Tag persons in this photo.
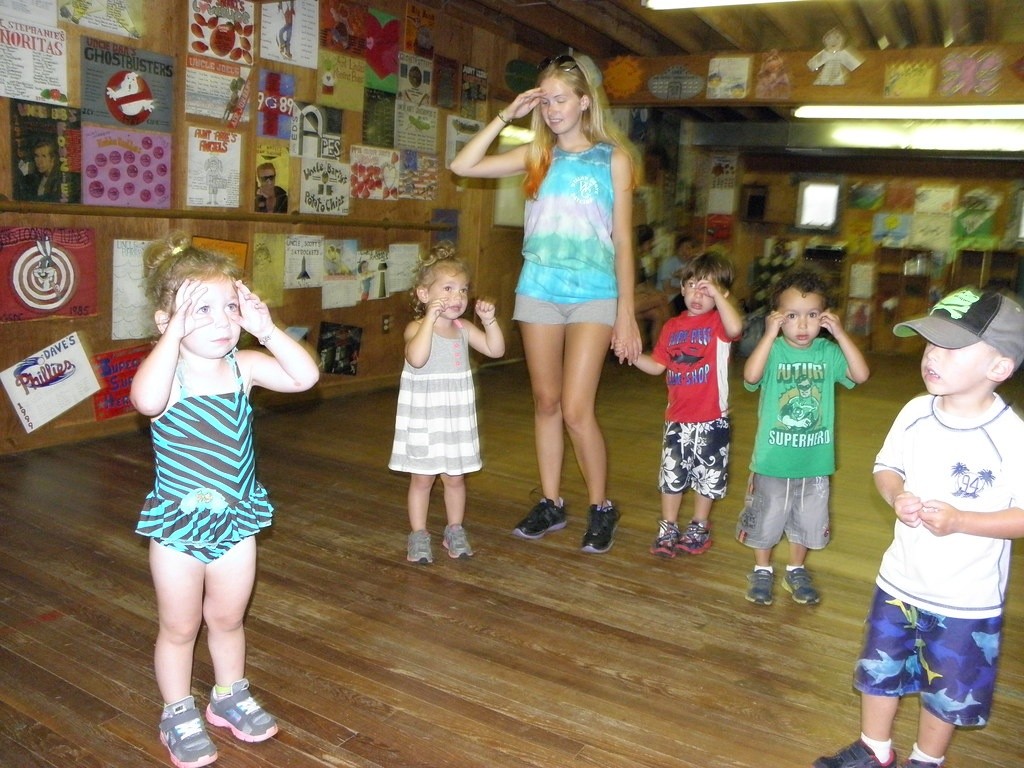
[18,139,81,203]
[734,271,869,608]
[633,224,671,349]
[449,47,642,553]
[813,286,1024,768]
[387,239,505,563]
[614,250,742,557]
[131,234,319,768]
[657,235,699,317]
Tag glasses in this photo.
[538,55,585,80]
[262,175,274,180]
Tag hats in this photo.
[892,289,1024,366]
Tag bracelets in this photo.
[256,324,275,345]
[497,110,510,124]
[481,317,496,326]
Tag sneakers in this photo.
[811,738,897,768]
[676,519,712,554]
[205,678,278,742]
[650,519,679,557]
[745,570,773,606]
[582,500,620,553]
[782,568,821,605]
[159,696,218,768]
[407,529,433,564]
[513,489,567,539]
[443,524,474,559]
